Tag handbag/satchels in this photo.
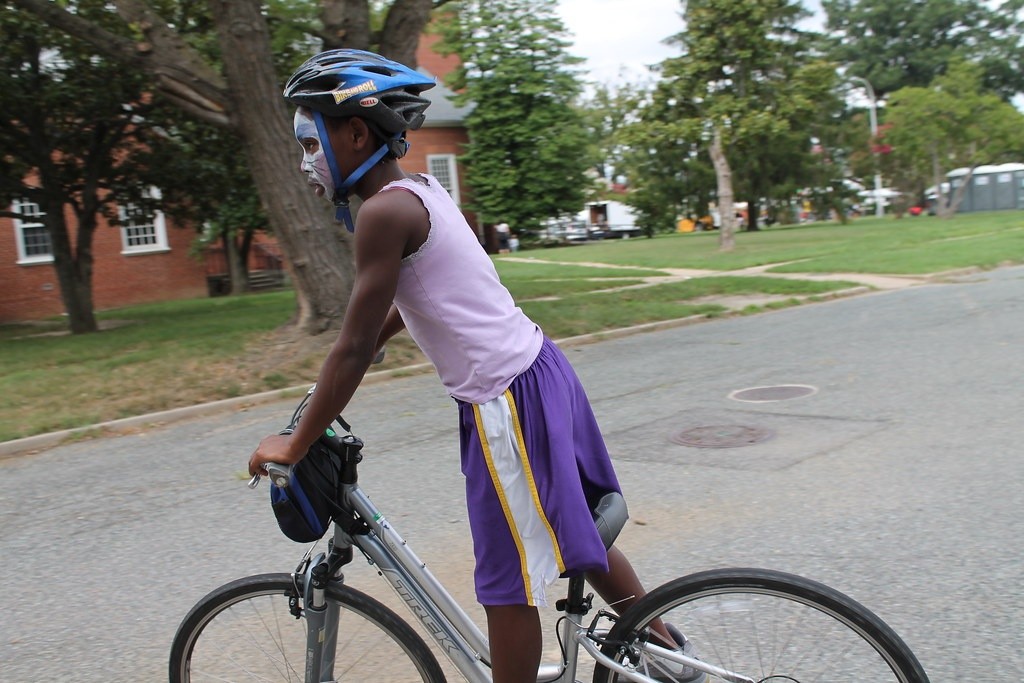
[270,417,372,544]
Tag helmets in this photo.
[281,48,436,139]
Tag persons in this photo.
[246,45,711,683]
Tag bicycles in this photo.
[167,384,929,683]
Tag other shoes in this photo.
[618,623,709,683]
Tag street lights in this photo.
[841,77,886,218]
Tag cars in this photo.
[565,223,588,240]
[854,187,898,216]
[832,179,865,198]
[588,224,644,241]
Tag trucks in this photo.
[584,201,643,230]
